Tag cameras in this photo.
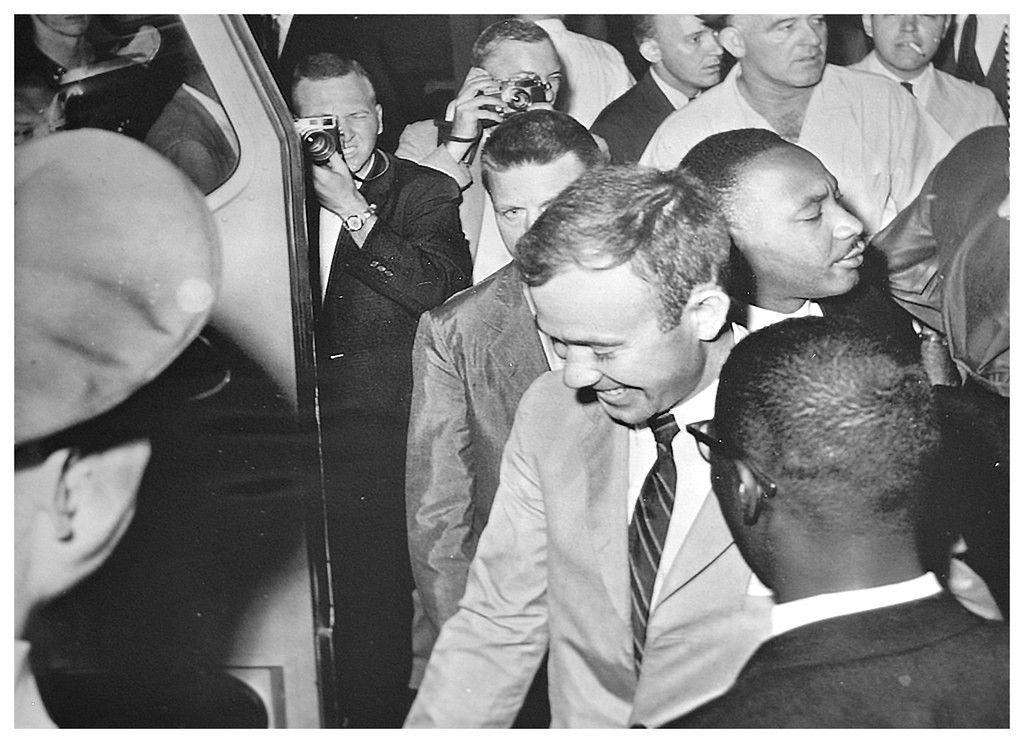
[478,77,540,125]
[293,115,341,163]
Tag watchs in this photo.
[342,204,376,231]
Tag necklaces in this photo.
[405,110,604,695]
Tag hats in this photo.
[15,127,231,444]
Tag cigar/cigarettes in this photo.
[909,42,925,57]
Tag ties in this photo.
[627,410,682,681]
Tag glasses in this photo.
[685,419,777,497]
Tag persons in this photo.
[636,15,957,245]
[285,51,472,729]
[141,40,239,195]
[14,129,234,729]
[674,128,921,366]
[868,124,1009,397]
[514,14,636,132]
[656,316,1010,729]
[840,13,1008,143]
[588,16,723,163]
[397,164,1004,729]
[395,19,613,285]
